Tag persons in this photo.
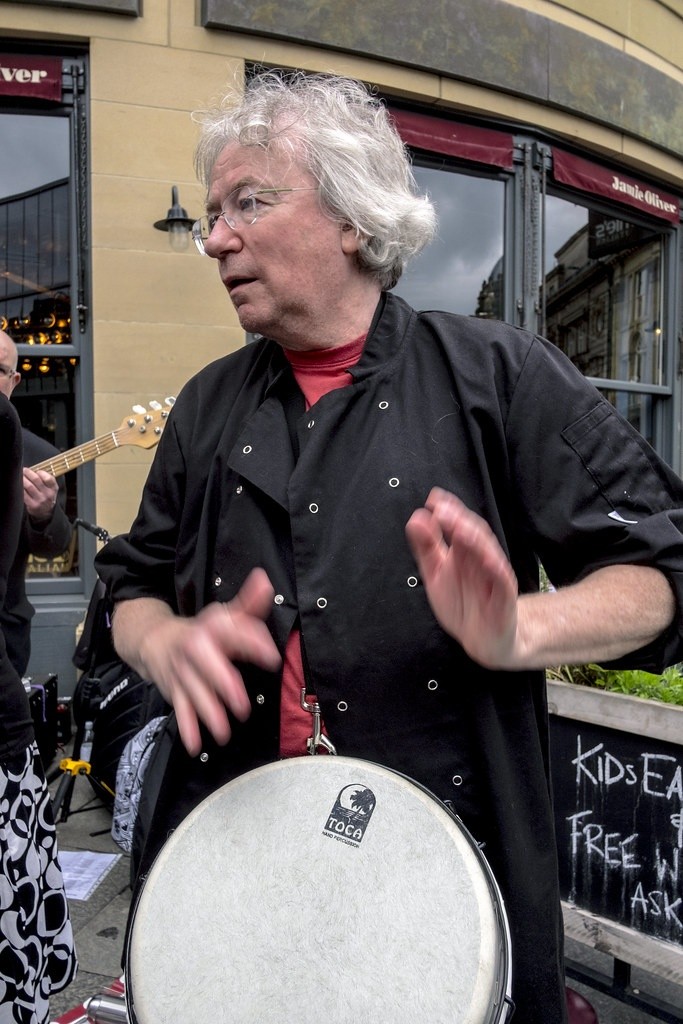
[69,64,683,1024]
[0,320,96,1014]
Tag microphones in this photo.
[76,518,108,541]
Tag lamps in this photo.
[154,186,197,253]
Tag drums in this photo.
[123,755,518,1024]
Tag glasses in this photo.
[190,186,319,256]
[0,364,15,377]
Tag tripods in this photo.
[46,579,118,825]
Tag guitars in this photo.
[23,394,180,479]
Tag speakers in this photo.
[19,671,57,772]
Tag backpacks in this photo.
[111,716,169,854]
